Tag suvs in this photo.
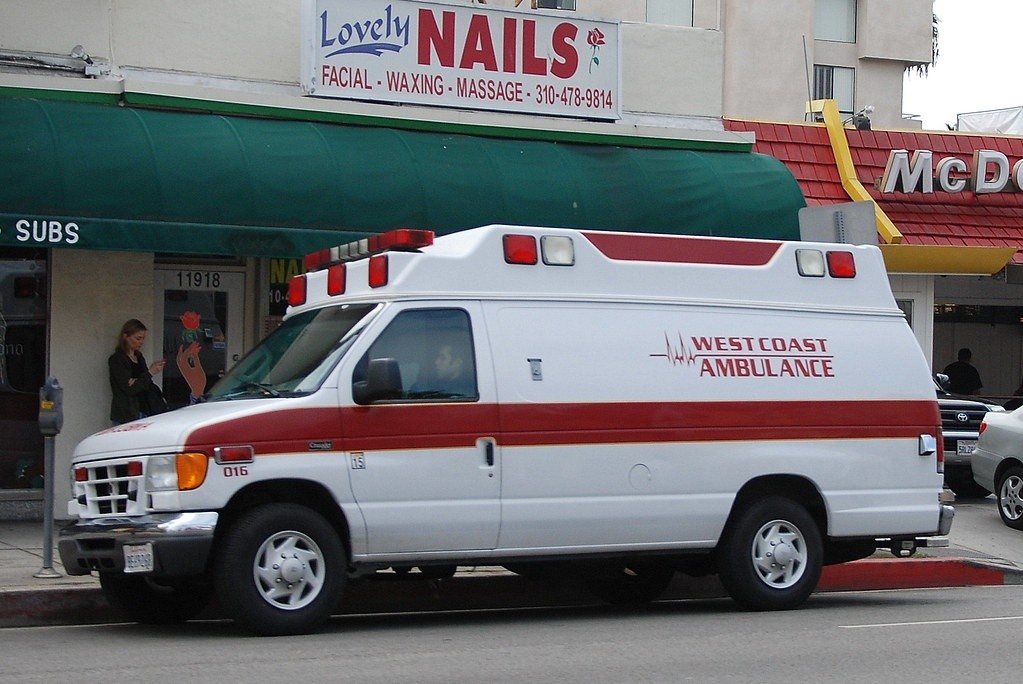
[931,372,1009,497]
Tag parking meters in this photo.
[34,375,66,579]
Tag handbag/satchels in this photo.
[139,384,167,417]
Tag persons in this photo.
[108,319,169,425]
[409,343,476,399]
[942,348,983,397]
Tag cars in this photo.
[969,403,1023,530]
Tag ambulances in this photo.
[60,221,956,638]
